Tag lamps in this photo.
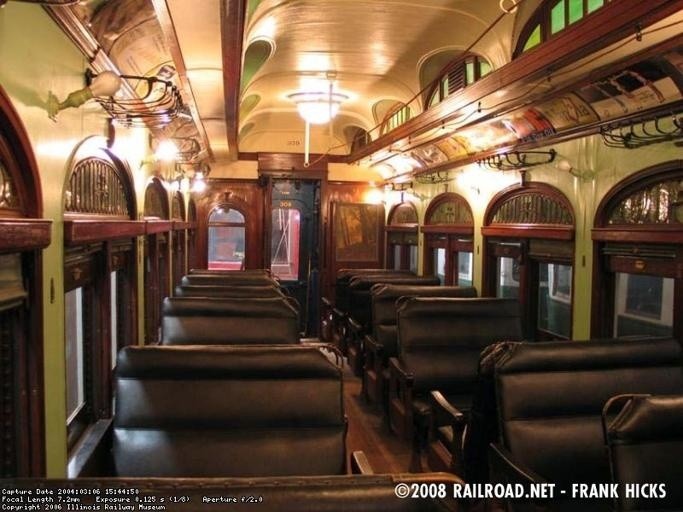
[47,71,120,122]
[406,188,425,200]
[287,91,347,125]
[141,142,178,167]
[557,161,591,182]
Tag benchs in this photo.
[0,270,683,512]
